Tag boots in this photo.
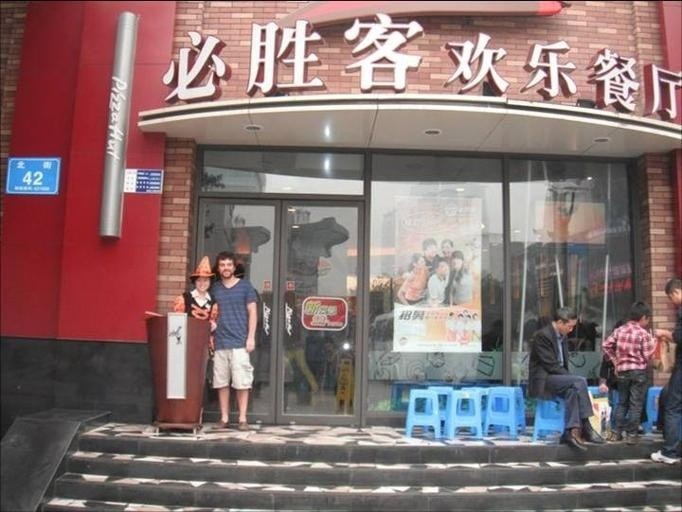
[610,429,641,444]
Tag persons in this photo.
[650,277,681,464]
[529,306,606,453]
[211,251,260,430]
[599,320,645,435]
[600,302,659,446]
[396,237,477,310]
[173,256,219,430]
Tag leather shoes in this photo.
[582,428,605,443]
[213,419,228,430]
[560,436,589,454]
[238,421,247,432]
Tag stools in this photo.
[642,388,663,434]
[406,386,619,441]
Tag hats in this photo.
[189,255,215,279]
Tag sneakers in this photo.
[651,447,679,464]
